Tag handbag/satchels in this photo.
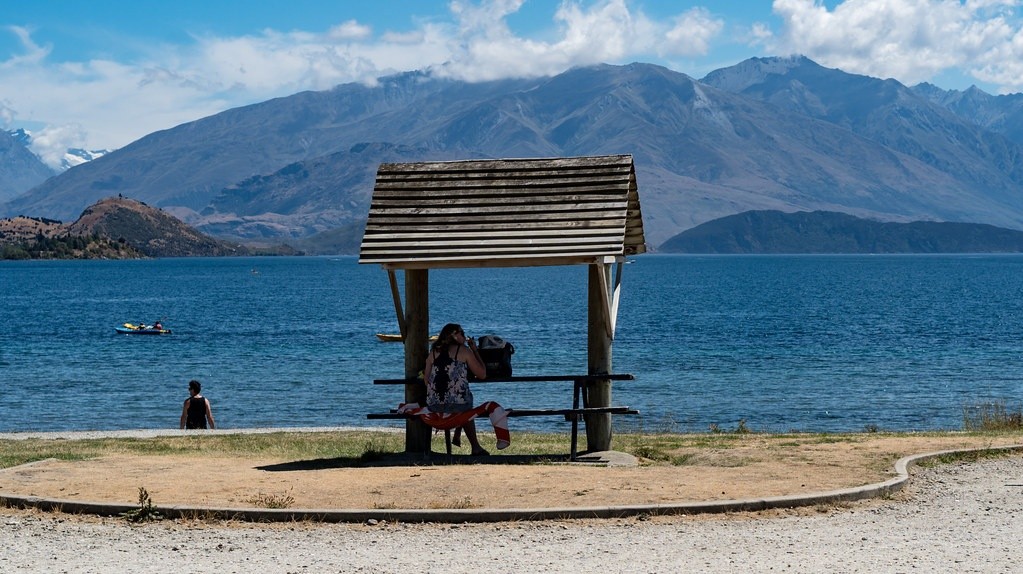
[467,335,515,379]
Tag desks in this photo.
[373,374,636,463]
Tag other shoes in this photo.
[451,439,461,447]
[470,450,489,456]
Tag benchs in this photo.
[366,406,629,421]
[388,408,640,415]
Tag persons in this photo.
[181,381,214,429]
[136,323,145,330]
[153,322,162,330]
[424,323,490,455]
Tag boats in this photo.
[114,324,171,335]
[377,333,439,341]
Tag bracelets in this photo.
[474,350,478,352]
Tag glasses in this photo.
[458,330,464,336]
